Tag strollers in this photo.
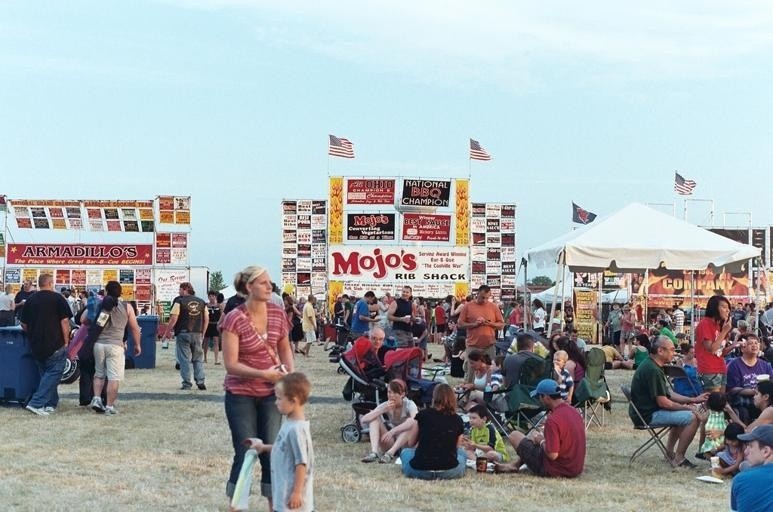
[335,332,444,444]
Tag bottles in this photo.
[484,383,492,402]
[162,336,168,352]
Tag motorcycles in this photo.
[56,319,81,385]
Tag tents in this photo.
[524,202,761,339]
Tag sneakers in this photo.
[25,405,55,417]
[198,383,206,390]
[181,386,191,390]
[91,396,105,412]
[360,451,378,462]
[104,407,119,415]
[380,451,394,463]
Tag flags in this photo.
[571,201,596,226]
[673,170,695,195]
[327,134,354,158]
[469,139,493,161]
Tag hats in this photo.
[621,304,630,311]
[737,425,773,446]
[181,282,193,290]
[529,379,561,399]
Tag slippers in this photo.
[661,452,700,469]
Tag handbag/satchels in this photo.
[67,324,88,359]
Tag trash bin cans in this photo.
[0,327,41,407]
[126,315,158,369]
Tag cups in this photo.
[476,456,487,472]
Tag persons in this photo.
[0,279,247,415]
[730,423,773,512]
[604,294,771,480]
[269,282,588,479]
[239,372,314,512]
[214,265,293,512]
